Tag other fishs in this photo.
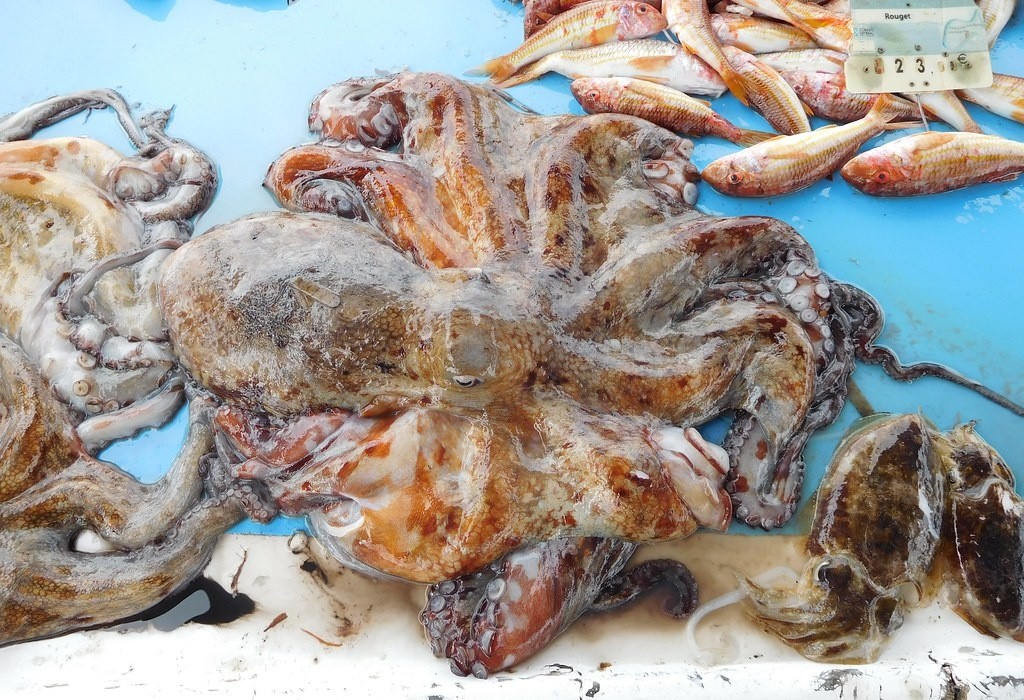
[473,1,1024,198]
